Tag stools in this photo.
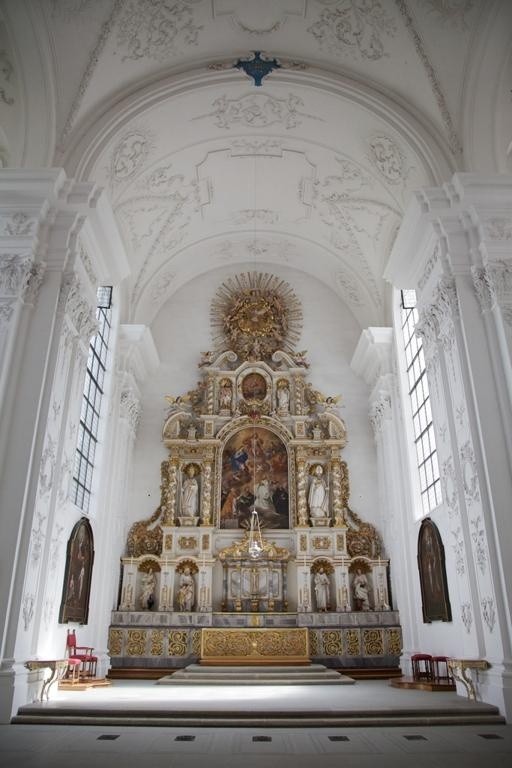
[431,656,454,684]
[411,654,433,683]
[67,658,81,679]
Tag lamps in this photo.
[247,340,264,558]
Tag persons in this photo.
[314,566,331,614]
[137,566,157,611]
[351,567,373,612]
[220,432,281,518]
[177,469,199,515]
[217,383,233,409]
[420,528,441,600]
[275,383,288,412]
[177,568,196,613]
[63,524,91,613]
[306,465,331,517]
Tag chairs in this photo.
[68,629,98,678]
